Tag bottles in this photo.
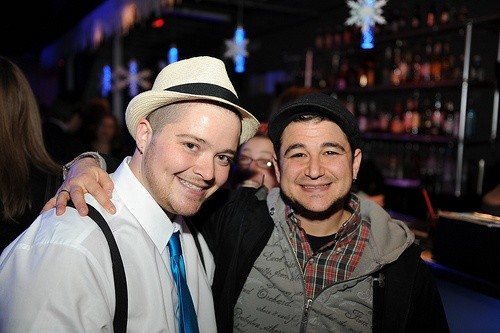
[310,7,483,191]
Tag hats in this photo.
[267,92,362,141]
[124,56,261,146]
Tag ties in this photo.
[167,230,200,333]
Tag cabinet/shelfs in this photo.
[304,18,500,223]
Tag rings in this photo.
[59,188,71,197]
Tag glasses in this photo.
[237,154,274,169]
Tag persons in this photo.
[229,130,279,195]
[0,55,263,333]
[38,84,124,173]
[38,83,452,333]
[0,57,65,253]
